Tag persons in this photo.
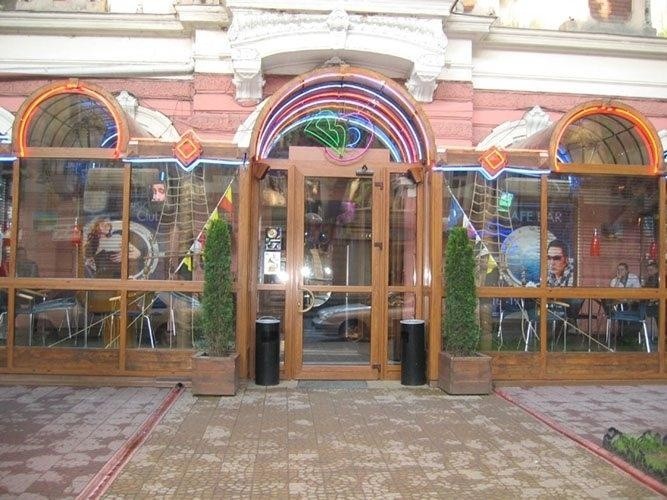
[546,238,574,287]
[149,183,166,202]
[609,262,641,288]
[84,213,141,280]
[642,262,659,288]
[14,247,39,278]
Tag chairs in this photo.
[491,285,659,352]
[0,288,155,347]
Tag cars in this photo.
[313,291,404,344]
[15,291,200,350]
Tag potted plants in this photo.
[190,217,240,398]
[438,228,496,397]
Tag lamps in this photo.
[589,206,601,255]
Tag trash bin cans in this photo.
[400,319,426,385]
[255,315,280,386]
